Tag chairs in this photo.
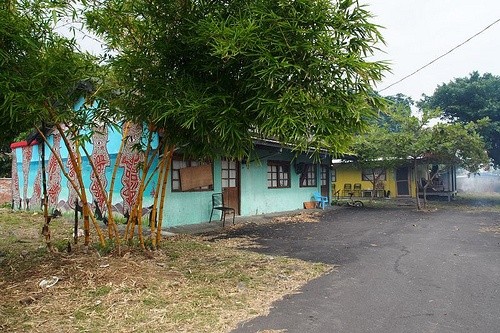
[314,191,329,210]
[208,192,235,226]
[341,183,352,197]
[353,183,362,196]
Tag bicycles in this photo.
[333,190,364,207]
